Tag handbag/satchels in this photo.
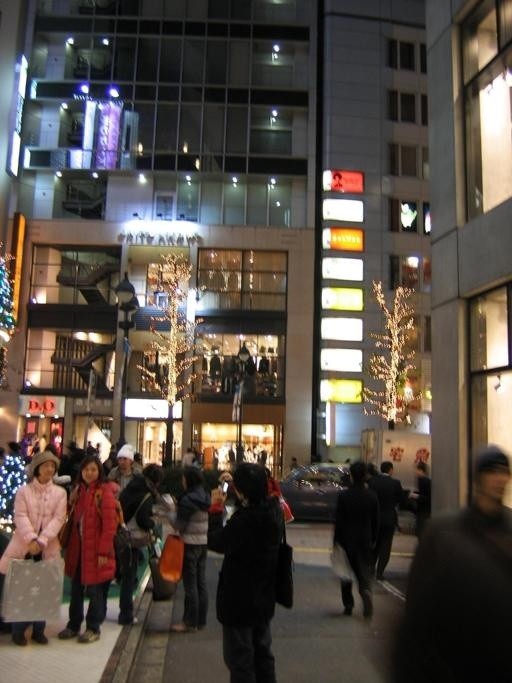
[113,523,137,586]
[277,541,293,608]
[1,557,65,622]
[159,533,184,583]
[126,517,155,547]
[58,510,72,546]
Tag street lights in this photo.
[115,272,144,446]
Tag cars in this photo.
[274,461,352,520]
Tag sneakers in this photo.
[58,627,79,638]
[78,628,100,642]
[12,634,26,644]
[170,623,197,632]
[30,631,47,643]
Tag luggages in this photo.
[147,544,177,597]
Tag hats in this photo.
[116,443,134,460]
[31,450,59,475]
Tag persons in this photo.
[333,460,407,619]
[414,460,433,537]
[0,439,209,647]
[207,461,285,682]
[289,457,299,471]
[394,446,511,681]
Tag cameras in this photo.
[221,480,229,496]
